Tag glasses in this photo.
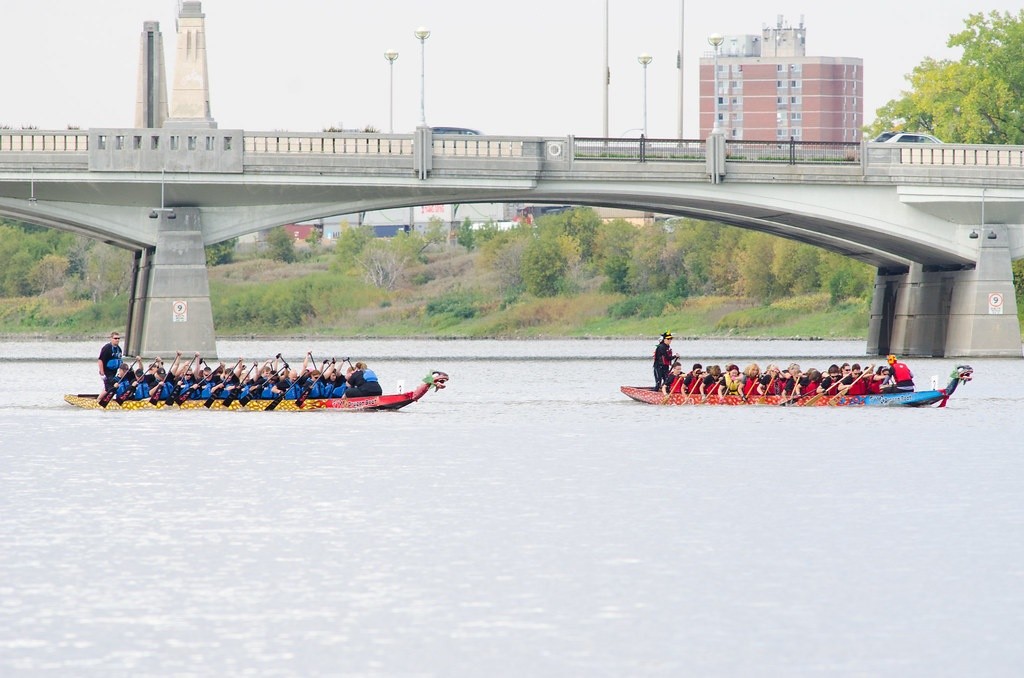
[854,372,861,376]
[883,373,891,377]
[844,368,851,372]
[794,375,798,380]
[112,337,121,341]
[185,373,194,378]
[830,374,839,379]
[314,376,318,379]
[203,372,209,378]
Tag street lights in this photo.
[708,33,725,134]
[638,53,653,147]
[385,48,399,135]
[415,27,431,125]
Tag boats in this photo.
[620,365,974,408]
[64,370,449,412]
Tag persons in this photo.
[112,351,382,400]
[883,355,915,393]
[97,332,122,400]
[653,332,680,392]
[662,362,889,403]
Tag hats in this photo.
[660,330,677,339]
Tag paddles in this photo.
[98,348,356,412]
[659,353,878,408]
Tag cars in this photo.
[432,127,480,135]
[873,131,943,143]
